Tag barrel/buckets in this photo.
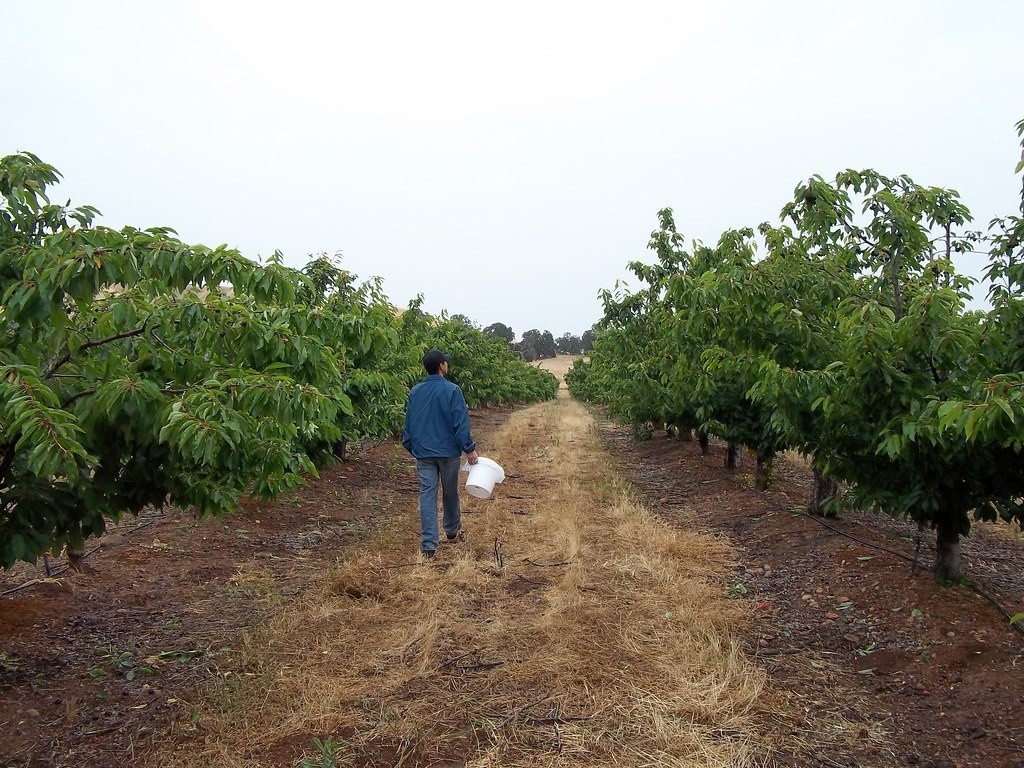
[465,458,504,499]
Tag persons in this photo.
[400,350,478,557]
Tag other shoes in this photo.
[447,521,461,541]
[422,550,435,558]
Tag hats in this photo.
[422,350,450,372]
